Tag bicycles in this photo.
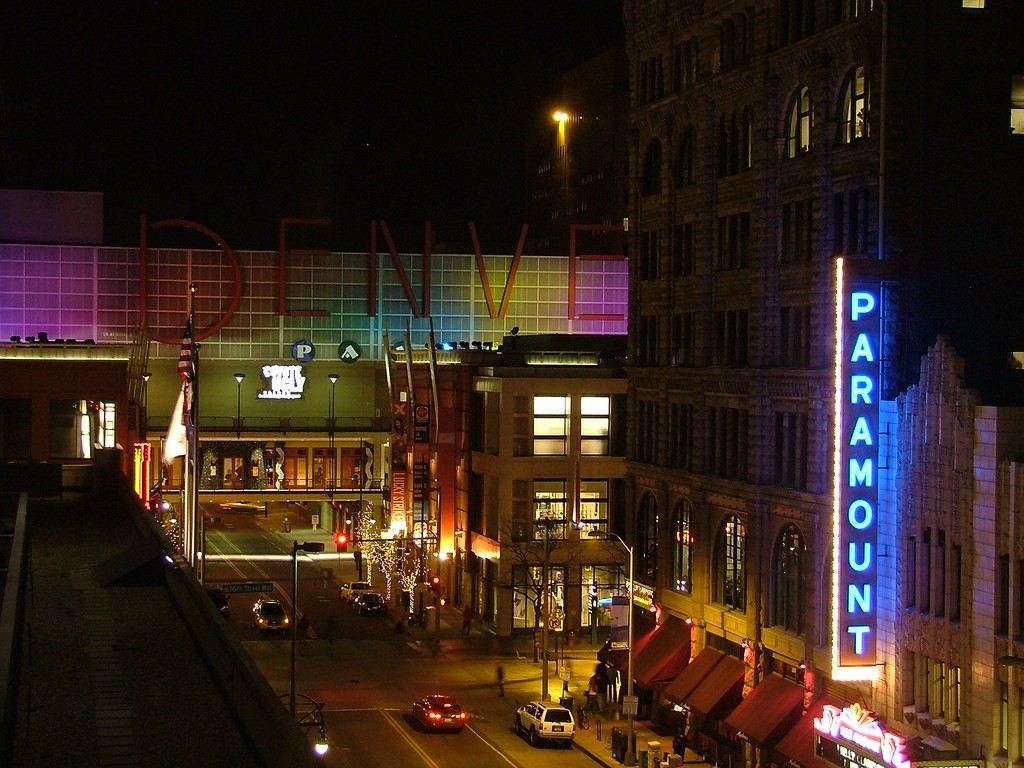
[574,704,590,731]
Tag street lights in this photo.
[327,373,341,436]
[233,372,246,439]
[288,539,326,721]
[140,370,153,437]
[587,528,637,766]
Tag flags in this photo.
[164,309,198,464]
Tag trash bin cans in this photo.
[638,749,648,768]
[648,740,662,768]
[612,726,636,763]
[558,697,574,713]
[564,629,578,647]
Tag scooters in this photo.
[407,609,430,628]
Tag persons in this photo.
[536,570,540,579]
[314,466,323,484]
[297,612,309,644]
[354,550,362,570]
[672,728,688,763]
[621,586,629,599]
[394,418,406,436]
[595,663,619,712]
[495,666,505,697]
[515,598,525,617]
[460,606,473,635]
[393,617,408,641]
[540,511,598,532]
[556,570,561,580]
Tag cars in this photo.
[220,501,266,514]
[413,694,466,734]
[340,581,375,605]
[252,597,291,635]
[353,593,388,616]
[205,588,232,621]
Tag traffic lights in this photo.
[433,576,441,588]
[335,532,349,553]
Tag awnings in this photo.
[597,612,849,768]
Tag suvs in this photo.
[515,701,576,748]
